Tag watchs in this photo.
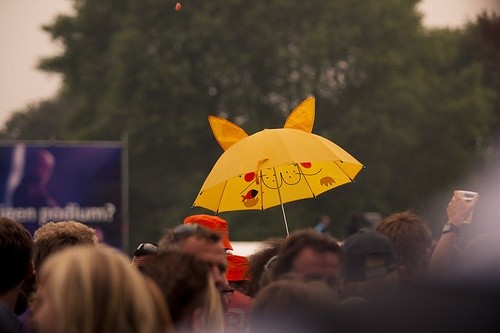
[440,223,460,235]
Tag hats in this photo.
[342,229,396,279]
[225,254,252,281]
[184,215,233,251]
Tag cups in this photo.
[454,190,476,225]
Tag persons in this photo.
[10,146,61,207]
[0,189,500,333]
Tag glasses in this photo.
[137,243,157,253]
[220,288,234,296]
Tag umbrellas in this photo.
[192,97,363,237]
